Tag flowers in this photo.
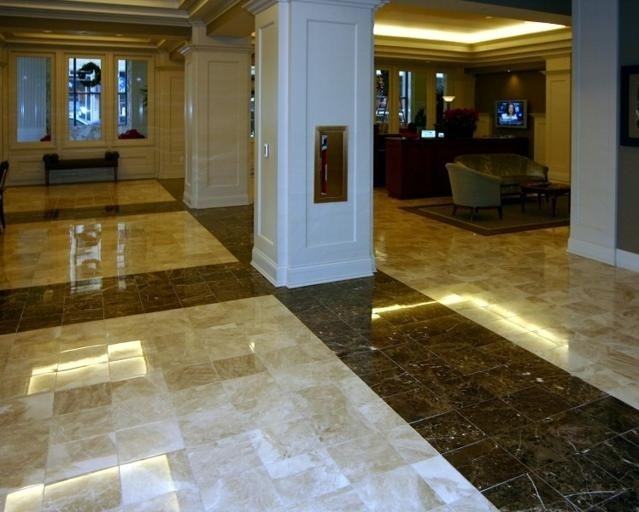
[442,108,480,122]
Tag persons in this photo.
[501,103,518,121]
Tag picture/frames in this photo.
[620,65,639,146]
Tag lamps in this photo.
[442,95,456,110]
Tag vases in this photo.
[447,119,474,139]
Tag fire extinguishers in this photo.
[321,133,329,196]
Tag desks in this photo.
[45,159,118,186]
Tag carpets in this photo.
[397,193,570,236]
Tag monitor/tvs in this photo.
[493,99,527,128]
[420,129,437,138]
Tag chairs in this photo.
[444,153,548,220]
[0,160,10,229]
